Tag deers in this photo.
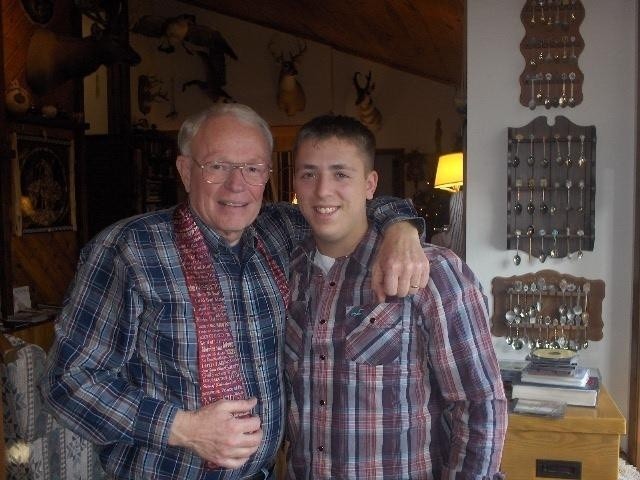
[352,70,384,132]
[273,35,308,118]
[24,0,142,96]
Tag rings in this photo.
[410,285,419,288]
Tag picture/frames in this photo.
[9,132,80,238]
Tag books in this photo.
[17,309,49,323]
[520,361,591,387]
[511,372,600,407]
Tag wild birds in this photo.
[157,11,197,53]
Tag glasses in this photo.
[189,155,273,187]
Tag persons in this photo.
[39,99,432,478]
[278,114,509,480]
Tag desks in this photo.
[488,387,630,479]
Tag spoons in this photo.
[523,0,578,111]
[504,279,592,351]
[511,134,587,265]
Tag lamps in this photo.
[431,151,464,194]
